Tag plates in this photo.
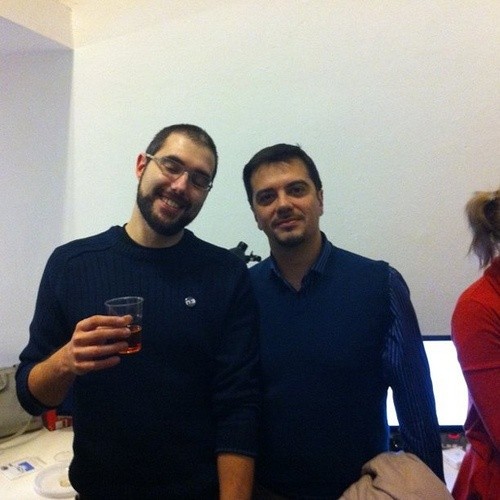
[34,463,80,497]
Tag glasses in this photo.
[145,153,213,192]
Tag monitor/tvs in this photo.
[386,335,469,434]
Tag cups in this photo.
[105,296,144,354]
[54,451,74,487]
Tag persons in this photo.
[451,190,500,500]
[14,123,262,500]
[243,144,446,500]
